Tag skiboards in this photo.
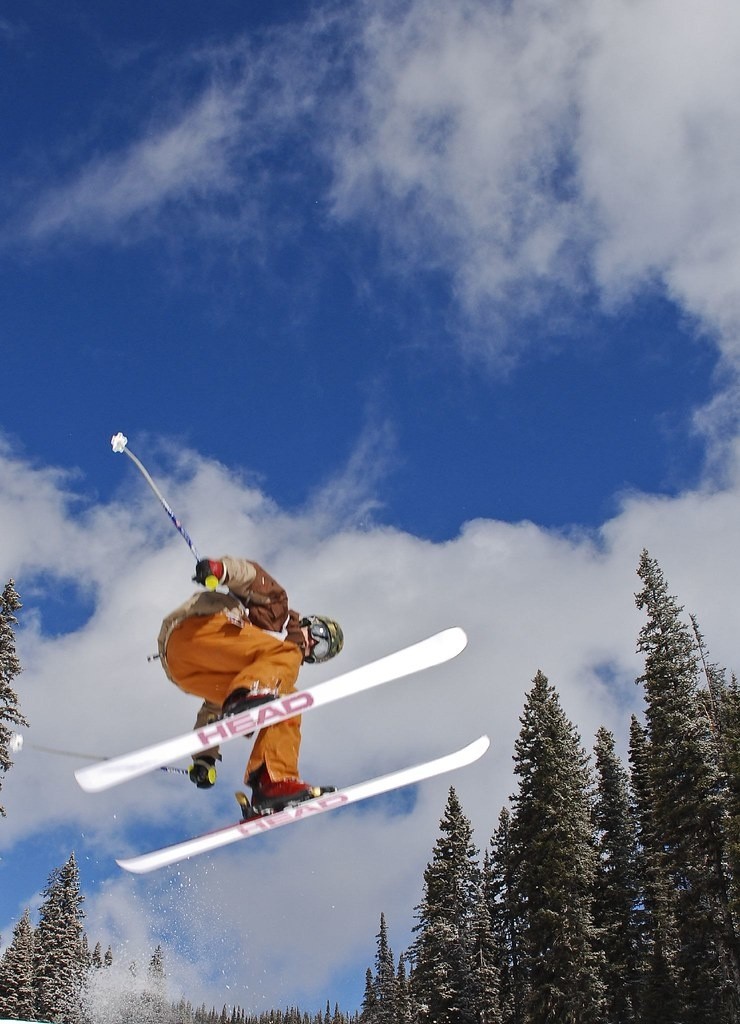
[72,624,491,876]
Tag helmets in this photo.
[300,614,343,663]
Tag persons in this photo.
[156,557,345,819]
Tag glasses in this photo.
[311,622,331,660]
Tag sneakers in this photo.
[222,679,281,719]
[250,766,311,814]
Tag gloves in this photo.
[188,755,217,789]
[191,559,227,586]
